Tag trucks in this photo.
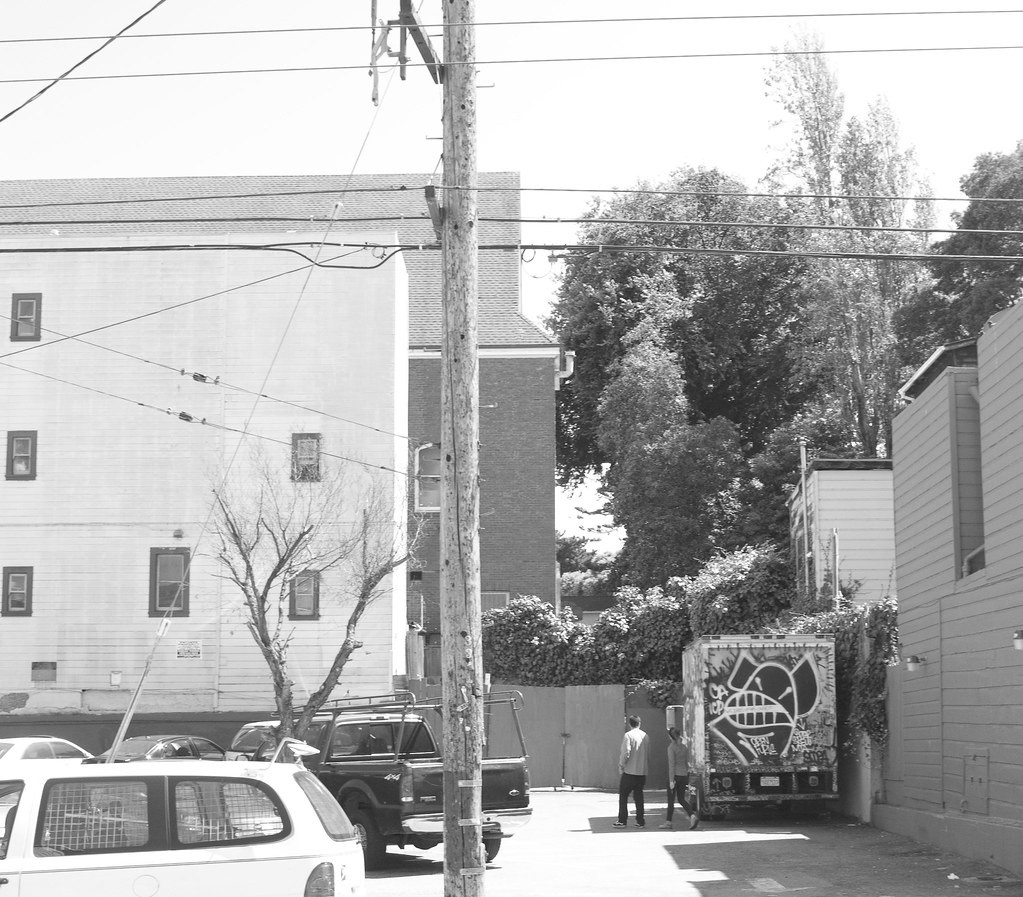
[665,632,839,819]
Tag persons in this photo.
[659,727,699,829]
[612,715,649,828]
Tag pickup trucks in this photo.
[235,689,534,870]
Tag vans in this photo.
[222,721,293,762]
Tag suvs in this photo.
[0,765,367,897]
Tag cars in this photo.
[0,736,94,765]
[79,733,225,796]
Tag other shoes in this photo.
[689,817,699,829]
[659,821,672,829]
[634,823,645,829]
[612,821,626,827]
